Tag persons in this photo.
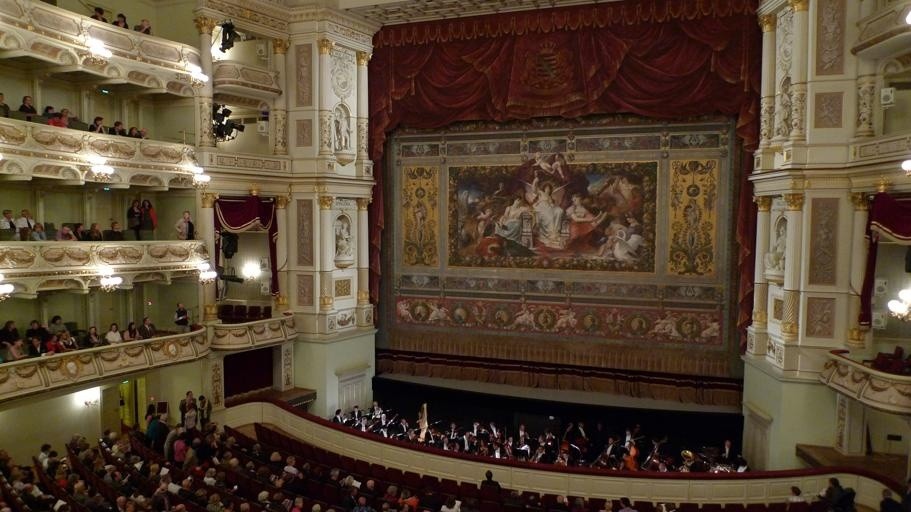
[112,13,128,29]
[90,7,107,22]
[0,86,150,138]
[0,301,153,366]
[173,302,192,332]
[2,200,158,241]
[175,210,194,239]
[473,151,649,265]
[338,111,353,151]
[133,19,151,34]
[774,225,786,270]
[338,221,352,256]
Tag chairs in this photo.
[0,322,155,362]
[383,467,539,510]
[0,107,141,139]
[1,423,381,512]
[221,304,271,323]
[543,492,819,512]
[0,227,154,241]
[872,344,910,375]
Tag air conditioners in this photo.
[879,85,897,106]
[256,43,267,57]
[873,276,890,293]
[256,120,268,133]
[872,309,888,329]
